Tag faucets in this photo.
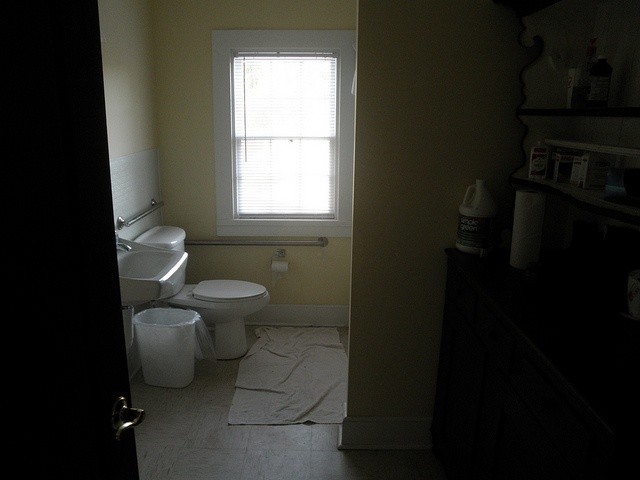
[118,242,132,252]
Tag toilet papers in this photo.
[272,260,289,273]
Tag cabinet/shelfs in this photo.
[445,2,640,375]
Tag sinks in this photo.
[117,250,189,301]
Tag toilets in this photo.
[133,225,271,360]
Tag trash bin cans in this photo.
[134,309,199,388]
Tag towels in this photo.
[230,326,347,424]
